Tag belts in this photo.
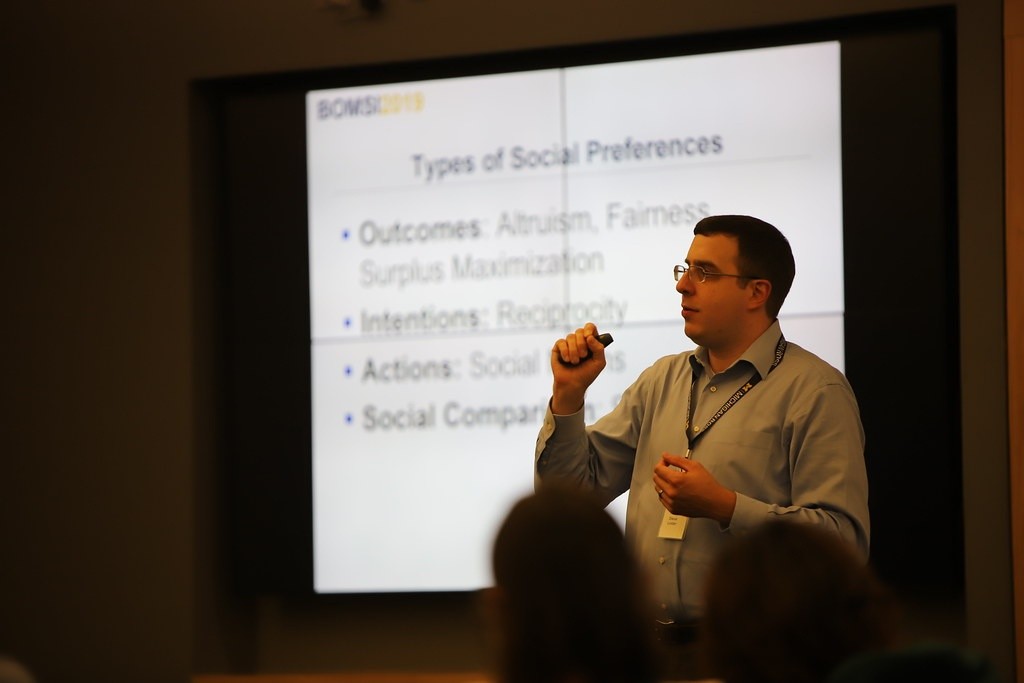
[649,618,705,648]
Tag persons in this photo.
[704,520,996,683]
[492,494,656,683]
[533,216,870,680]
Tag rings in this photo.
[659,490,663,499]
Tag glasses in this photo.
[674,265,761,284]
